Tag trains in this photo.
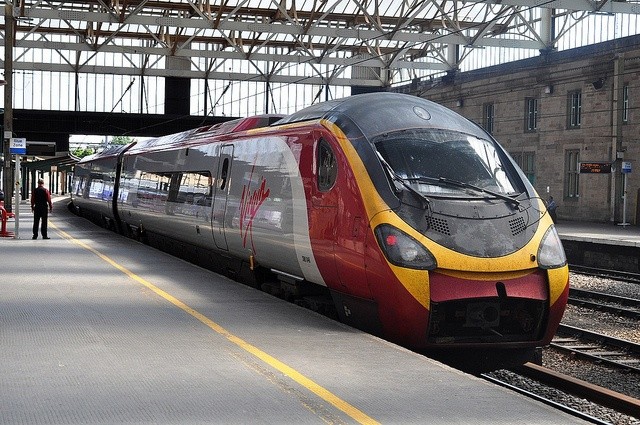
[71,92,569,353]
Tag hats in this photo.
[38,178,44,184]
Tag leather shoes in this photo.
[43,236,50,239]
[32,237,36,239]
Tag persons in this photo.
[32,178,52,239]
[547,195,556,225]
